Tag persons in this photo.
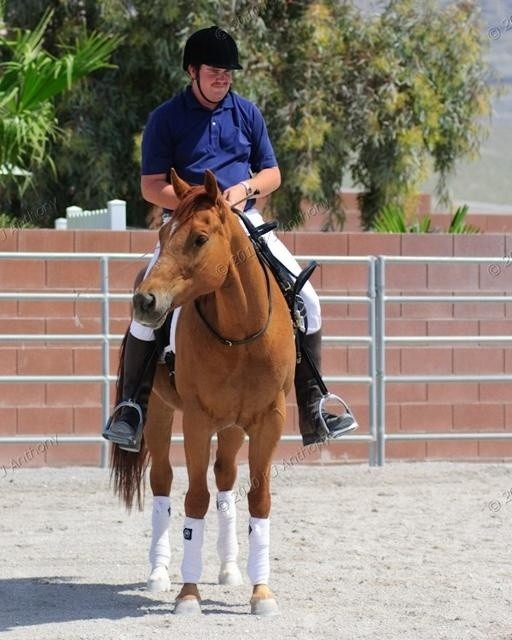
[105,22,355,445]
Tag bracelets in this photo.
[238,180,252,194]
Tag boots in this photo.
[108,330,160,453]
[294,327,354,447]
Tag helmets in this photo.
[183,25,243,70]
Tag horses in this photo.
[108,167,297,619]
